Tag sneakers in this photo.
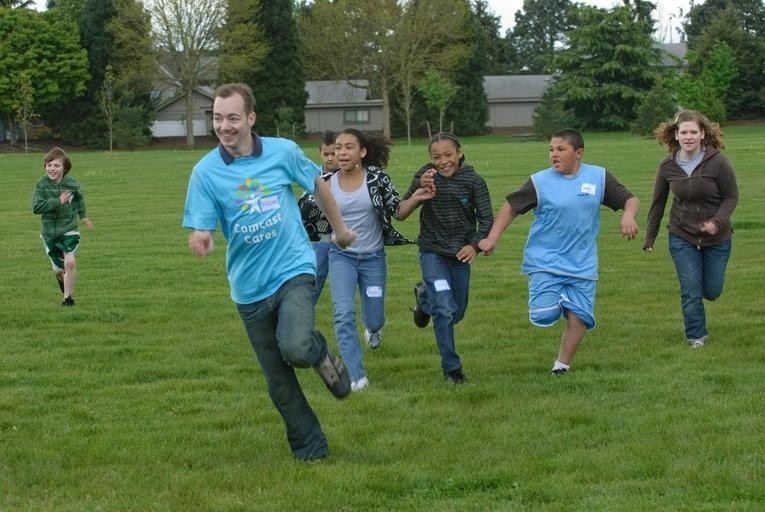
[313,348,351,398]
[350,377,370,391]
[62,295,74,306]
[443,364,468,384]
[364,329,383,350]
[56,273,64,293]
[413,282,430,327]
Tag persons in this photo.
[32,146,97,308]
[180,82,353,465]
[404,133,494,386]
[478,128,639,376]
[313,128,340,308]
[642,108,738,349]
[297,129,438,390]
